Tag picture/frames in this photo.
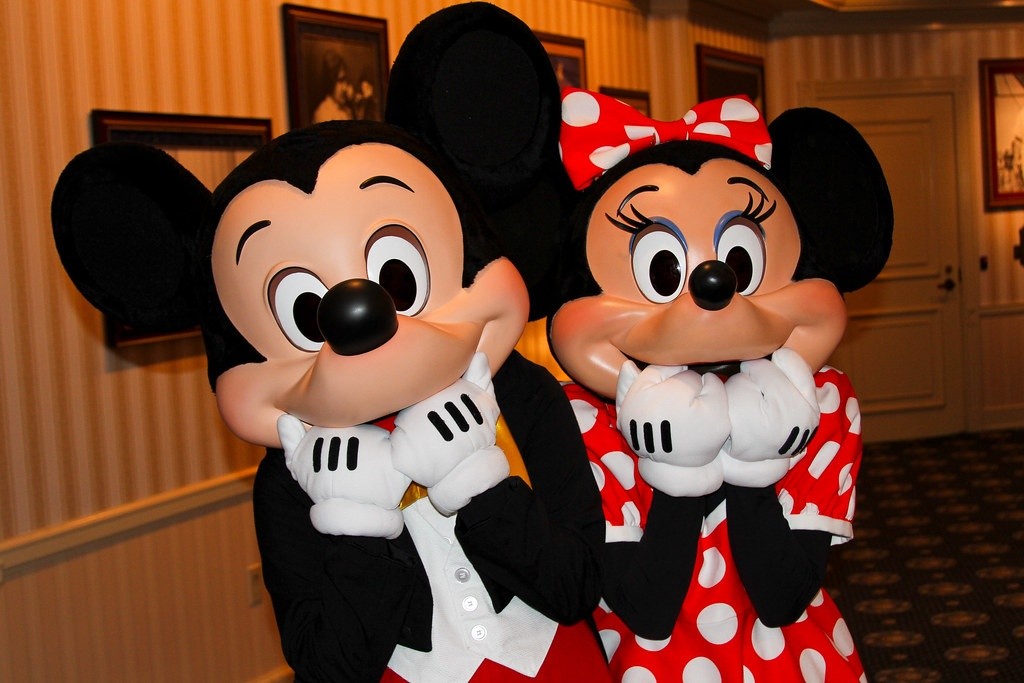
[600,85,651,118]
[531,29,587,90]
[281,3,390,133]
[978,57,1024,214]
[693,42,765,122]
[90,108,272,347]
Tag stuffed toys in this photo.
[51,1,896,683]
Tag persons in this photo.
[313,48,381,123]
